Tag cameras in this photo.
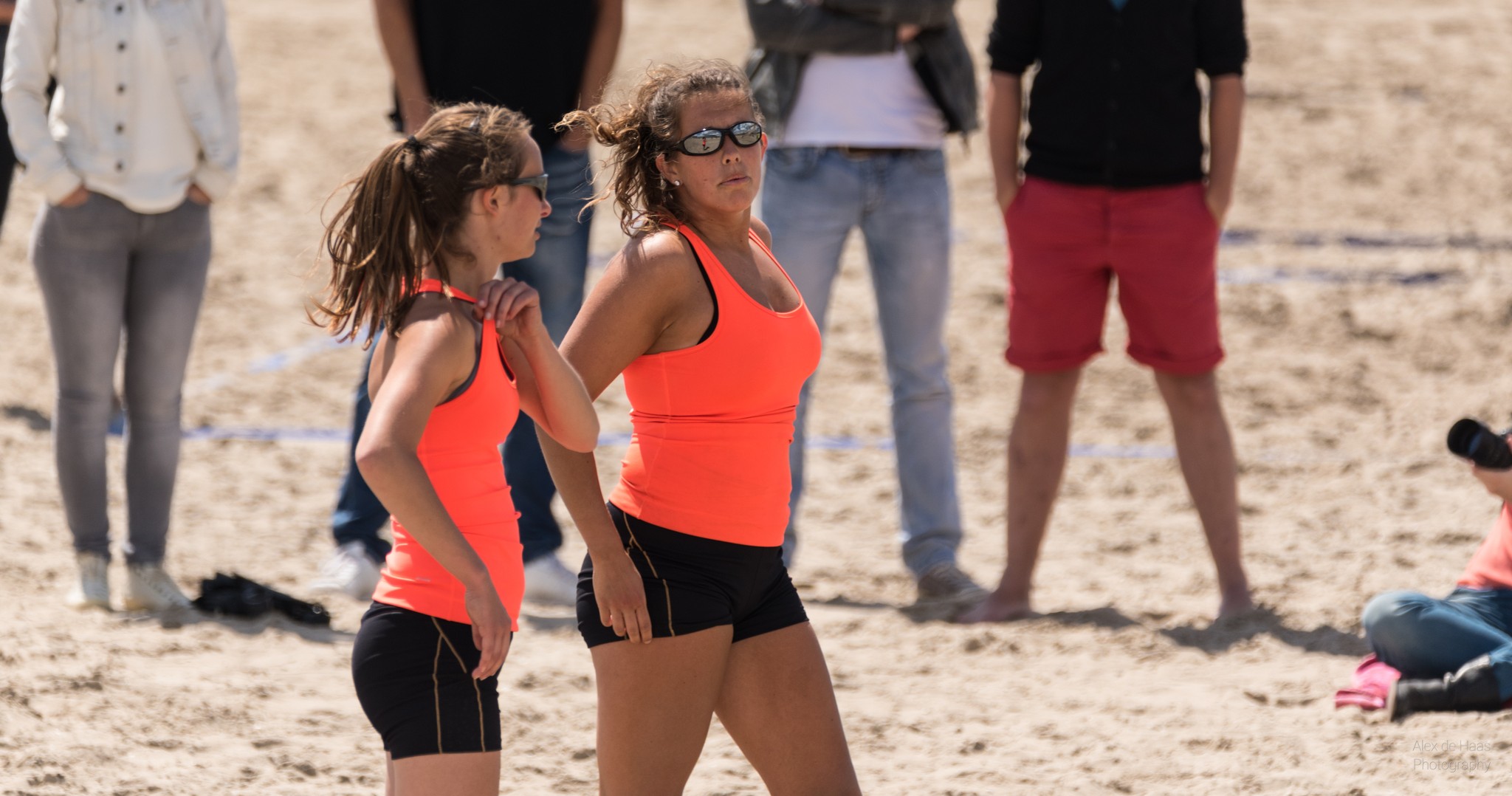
[1446,418,1512,470]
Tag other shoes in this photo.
[314,546,383,599]
[126,558,197,615]
[518,551,590,609]
[909,570,988,620]
[963,603,1036,623]
[1213,598,1287,634]
[70,553,115,610]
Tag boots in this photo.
[1387,652,1493,723]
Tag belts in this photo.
[831,143,913,158]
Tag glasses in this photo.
[671,121,762,155]
[490,174,547,202]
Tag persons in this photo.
[303,99,600,796]
[1362,429,1512,721]
[0,0,621,610]
[741,0,992,606]
[538,59,859,795]
[984,0,1285,619]
